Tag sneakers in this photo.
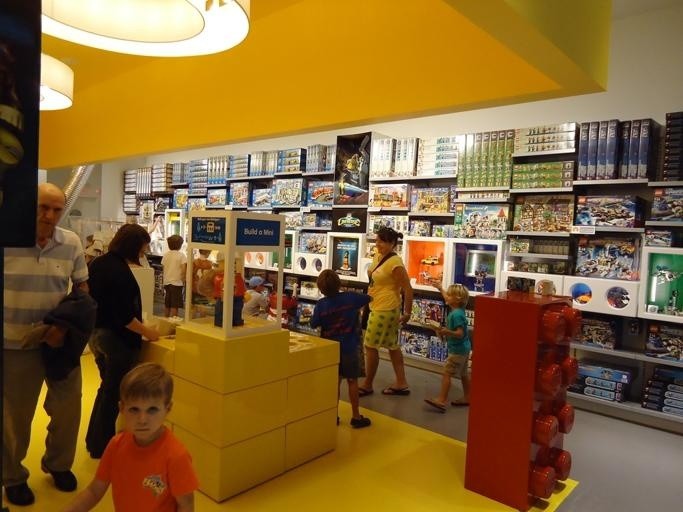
[337,416,339,424]
[426,398,449,412]
[351,417,372,429]
[452,397,469,407]
[214,321,223,327]
[233,319,244,325]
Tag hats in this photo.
[249,275,266,288]
[85,241,105,257]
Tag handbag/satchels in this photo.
[360,252,398,330]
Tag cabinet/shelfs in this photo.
[120,118,683,436]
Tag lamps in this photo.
[40,50,74,111]
[40,0,254,58]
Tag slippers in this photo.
[357,387,376,396]
[383,385,412,397]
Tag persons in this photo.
[309,270,375,429]
[353,226,410,398]
[56,361,196,512]
[243,274,270,322]
[192,246,219,315]
[3,181,90,506]
[156,233,189,319]
[270,282,296,328]
[421,275,474,411]
[84,223,166,462]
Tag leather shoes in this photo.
[4,483,36,507]
[85,441,96,448]
[40,453,78,492]
[89,448,103,456]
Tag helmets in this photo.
[216,251,241,261]
[572,284,592,298]
